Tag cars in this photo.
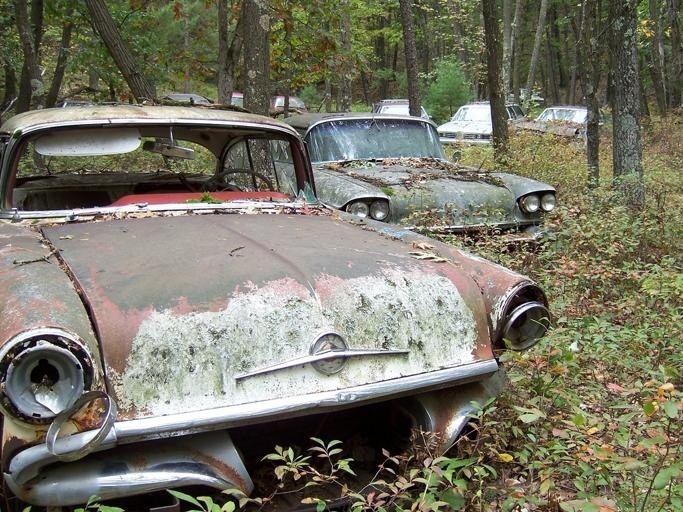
[234,112,557,252]
[0,104,551,512]
